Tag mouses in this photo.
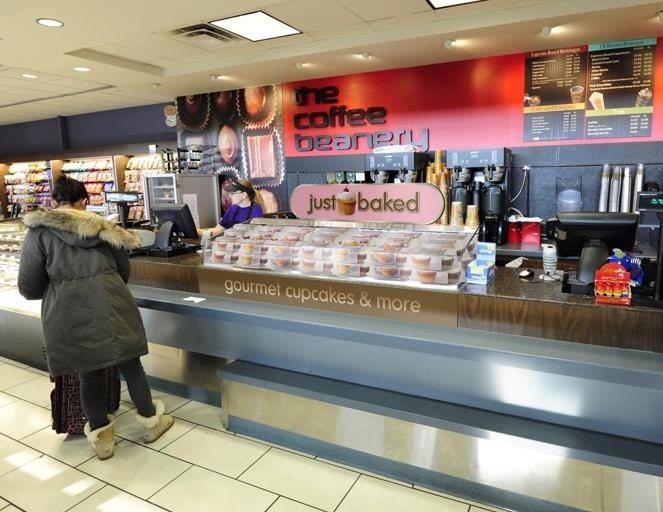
[519,270,534,277]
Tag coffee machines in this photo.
[365,151,425,183]
[446,146,510,243]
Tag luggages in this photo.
[50,365,120,435]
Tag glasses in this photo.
[230,191,241,195]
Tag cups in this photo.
[464,205,481,228]
[598,161,645,214]
[569,86,585,103]
[424,150,449,226]
[588,94,605,110]
[449,200,463,225]
[634,92,651,107]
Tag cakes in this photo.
[336,188,356,214]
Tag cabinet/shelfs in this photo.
[114,142,181,224]
[0,155,52,220]
[47,147,117,220]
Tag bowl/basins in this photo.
[527,96,540,106]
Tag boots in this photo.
[133,399,175,444]
[83,413,117,461]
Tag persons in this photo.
[195,177,262,240]
[16,174,174,463]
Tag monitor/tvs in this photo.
[147,204,200,257]
[557,212,639,294]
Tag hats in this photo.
[223,179,255,196]
